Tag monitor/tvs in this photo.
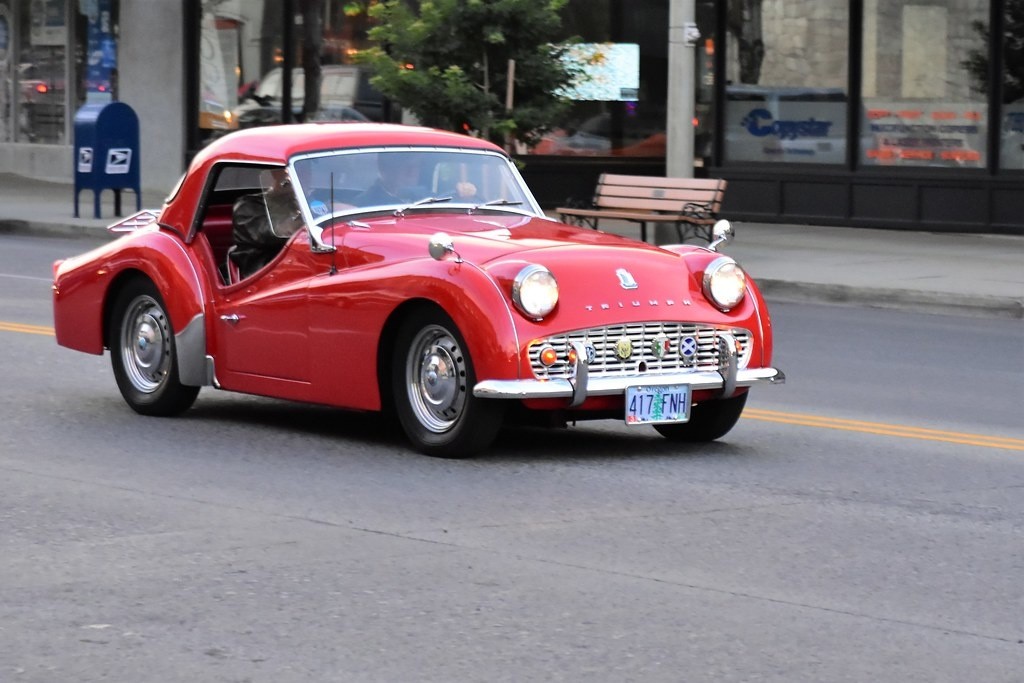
[549,43,639,101]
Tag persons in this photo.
[524,110,617,154]
[353,151,478,208]
[231,159,362,282]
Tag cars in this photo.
[17,60,115,114]
[231,63,404,124]
[534,107,668,158]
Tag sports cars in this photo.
[52,123,787,459]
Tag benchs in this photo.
[556,173,727,244]
